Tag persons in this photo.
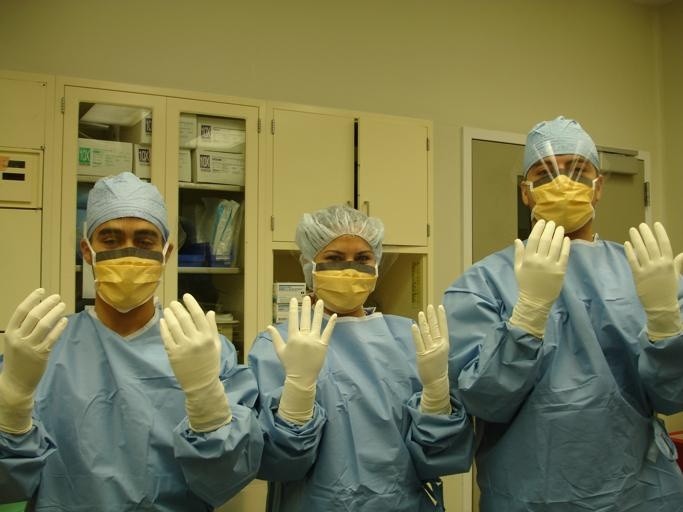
[234,202,479,510]
[442,112,682,512]
[0,170,268,509]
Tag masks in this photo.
[310,260,380,315]
[530,173,601,234]
[83,238,169,313]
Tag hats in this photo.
[86,171,170,243]
[522,115,601,180]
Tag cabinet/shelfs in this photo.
[260,101,433,329]
[441,475,464,512]
[54,74,267,511]
[1,72,54,358]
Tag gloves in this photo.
[158,292,233,433]
[267,295,338,426]
[410,303,453,416]
[0,287,69,436]
[508,219,571,340]
[623,221,683,343]
